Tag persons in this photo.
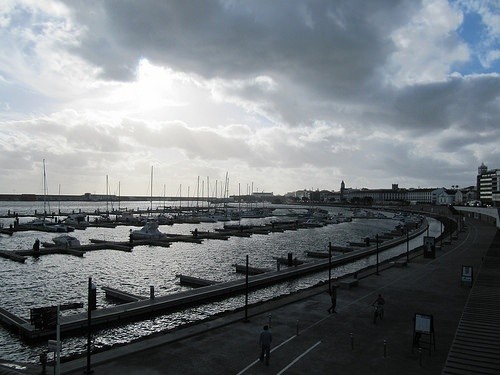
[327,288,337,314]
[259,325,272,361]
[372,294,385,320]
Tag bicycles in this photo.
[371,305,384,324]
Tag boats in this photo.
[300,212,351,228]
[392,213,406,222]
[51,234,80,246]
[133,223,166,240]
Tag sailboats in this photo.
[146,166,265,225]
[33,159,52,225]
[98,174,115,222]
